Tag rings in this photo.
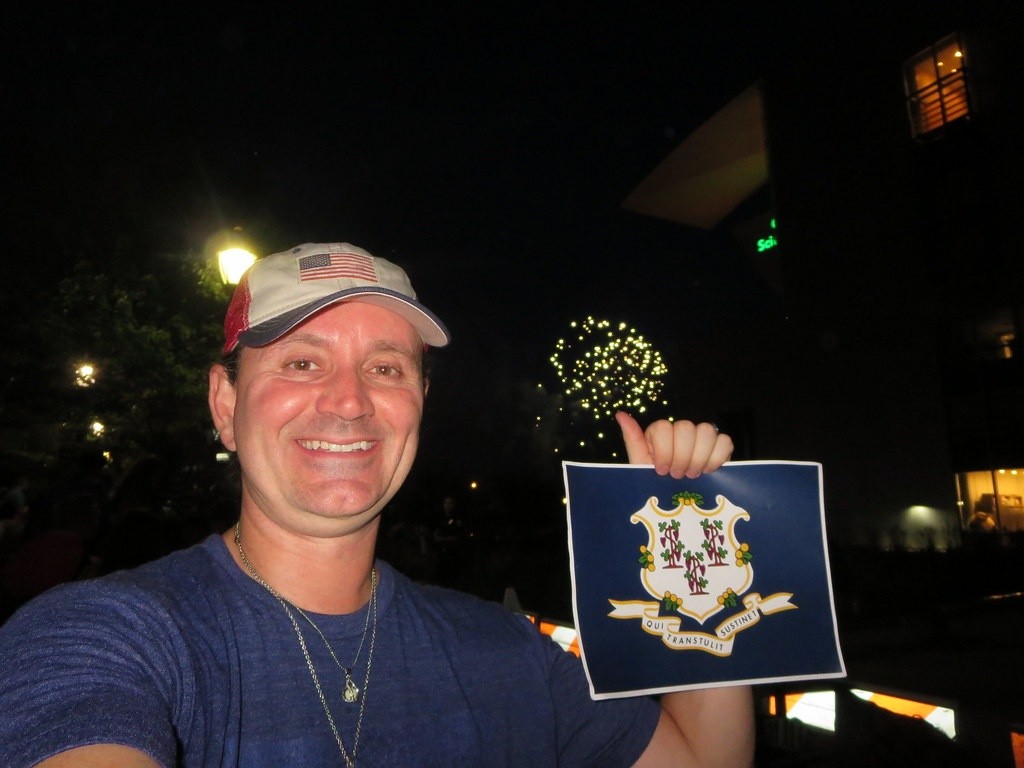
[711,421,720,433]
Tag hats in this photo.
[219,241,451,359]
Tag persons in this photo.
[0,241,755,768]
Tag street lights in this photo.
[216,225,258,383]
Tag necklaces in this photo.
[235,522,376,768]
[234,519,374,704]
